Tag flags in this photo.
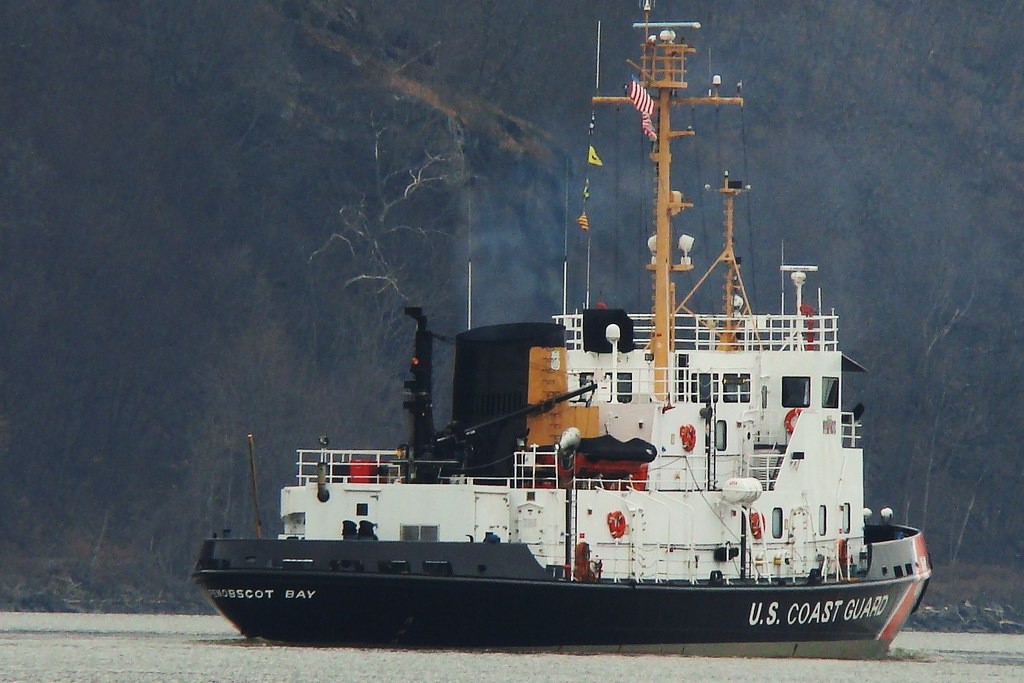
[575,212,589,231]
[587,146,602,166]
[630,80,654,116]
[587,112,596,134]
[583,177,590,200]
[642,114,657,141]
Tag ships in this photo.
[195,0,934,654]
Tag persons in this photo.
[389,444,407,484]
